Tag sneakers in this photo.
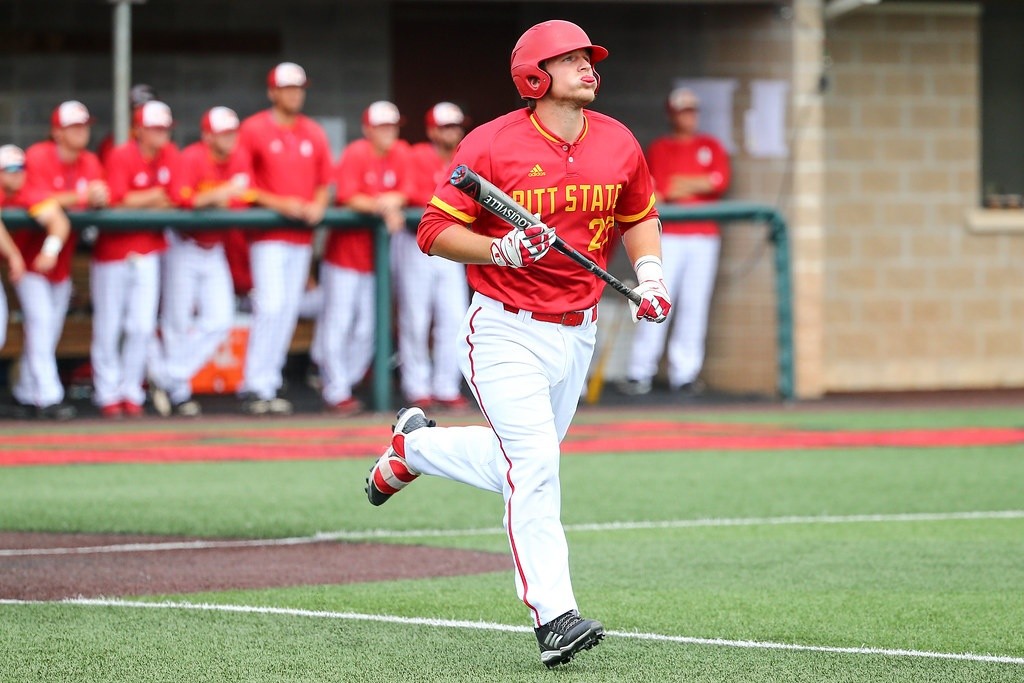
[534,610,605,669]
[364,406,436,507]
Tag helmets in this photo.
[509,19,609,101]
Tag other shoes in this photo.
[99,400,144,418]
[234,394,293,415]
[326,395,363,415]
[0,399,79,421]
[408,392,468,410]
[147,381,201,418]
[616,379,710,402]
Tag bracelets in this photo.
[41,235,63,258]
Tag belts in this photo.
[503,303,597,326]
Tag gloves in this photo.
[489,212,557,268]
[629,255,673,325]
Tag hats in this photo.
[265,61,310,89]
[423,100,472,129]
[130,83,157,106]
[360,100,407,127]
[0,144,26,171]
[132,99,176,131]
[199,106,242,137]
[48,99,96,129]
[666,86,702,113]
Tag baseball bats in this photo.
[448,162,641,307]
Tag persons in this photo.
[364,19,671,668]
[90,100,180,420]
[227,61,336,416]
[2,100,111,421]
[310,101,418,412]
[147,107,255,418]
[620,87,731,400]
[389,103,471,411]
[0,143,71,350]
